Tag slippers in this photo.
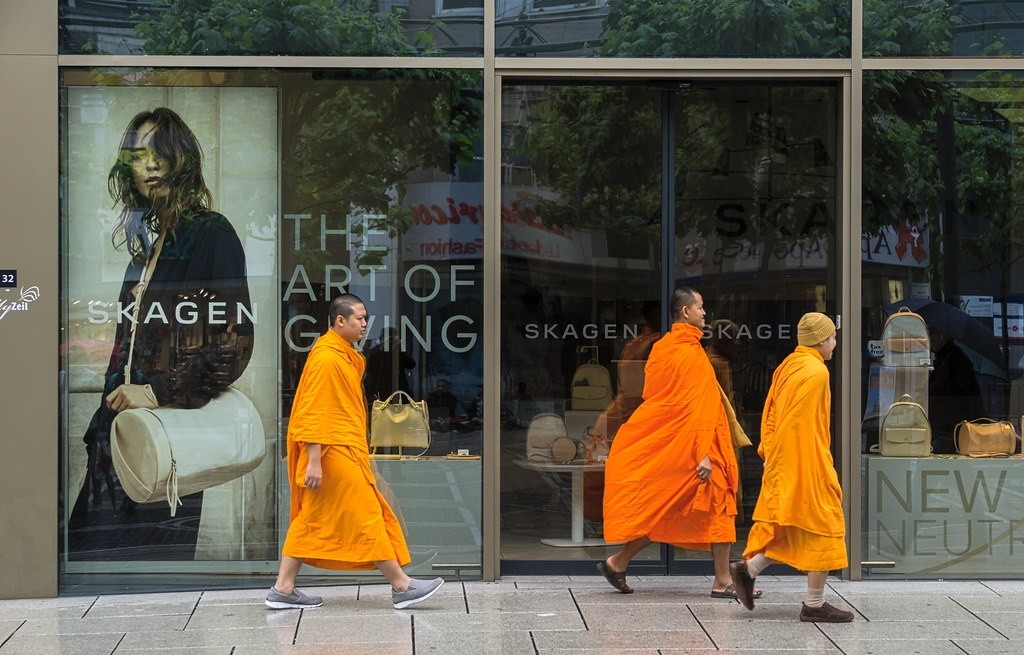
[711,584,762,598]
[596,561,634,593]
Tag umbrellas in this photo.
[885,298,1010,383]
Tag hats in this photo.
[797,313,835,347]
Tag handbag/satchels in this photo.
[564,410,608,464]
[578,425,609,461]
[954,417,1022,458]
[110,387,265,517]
[370,390,431,449]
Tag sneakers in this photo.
[799,601,854,623]
[392,577,444,609]
[729,560,756,610]
[265,586,324,608]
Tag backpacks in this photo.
[869,394,933,457]
[570,359,613,410]
[878,306,932,366]
[524,412,567,461]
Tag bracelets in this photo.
[143,385,158,406]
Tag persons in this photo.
[597,287,763,599]
[922,324,988,452]
[265,294,444,610]
[427,379,456,432]
[729,313,854,622]
[709,318,738,408]
[584,299,662,523]
[365,326,416,481]
[69,108,254,561]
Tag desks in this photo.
[280,454,481,567]
[861,454,1024,580]
[513,459,627,547]
